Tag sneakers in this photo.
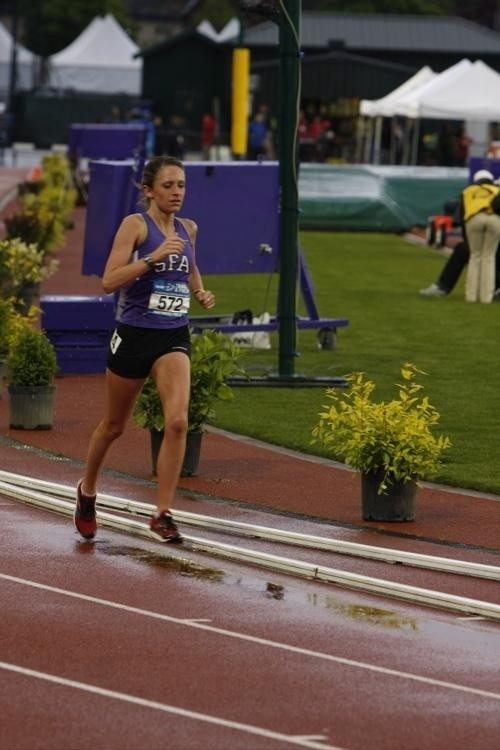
[74,476,97,539]
[149,509,184,543]
[419,283,445,296]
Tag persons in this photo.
[451,167,499,304]
[403,213,468,298]
[73,156,216,543]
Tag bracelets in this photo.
[142,254,157,269]
[193,289,207,295]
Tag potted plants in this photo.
[0,238,59,313]
[309,362,450,524]
[0,296,57,429]
[134,328,239,475]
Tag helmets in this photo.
[474,169,494,183]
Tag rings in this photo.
[207,298,211,302]
